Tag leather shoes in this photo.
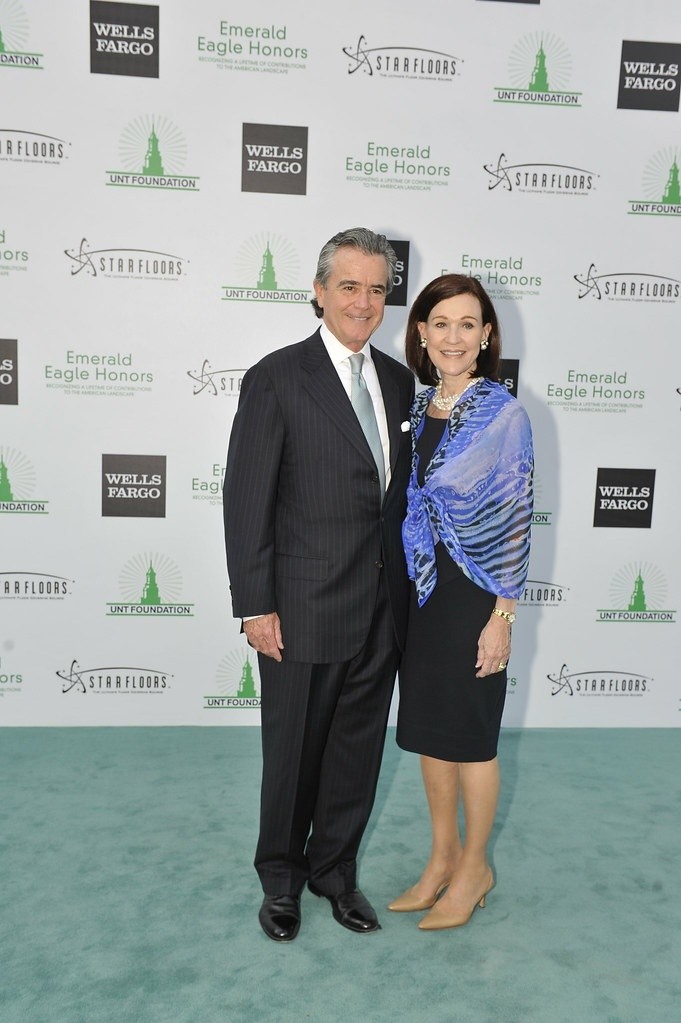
[308,881,380,933]
[259,892,303,941]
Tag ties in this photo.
[349,354,386,512]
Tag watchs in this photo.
[491,605,518,625]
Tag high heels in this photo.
[387,875,455,912]
[418,867,495,930]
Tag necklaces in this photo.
[429,373,483,413]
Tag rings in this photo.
[498,662,507,670]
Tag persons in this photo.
[389,274,535,932]
[221,227,416,941]
